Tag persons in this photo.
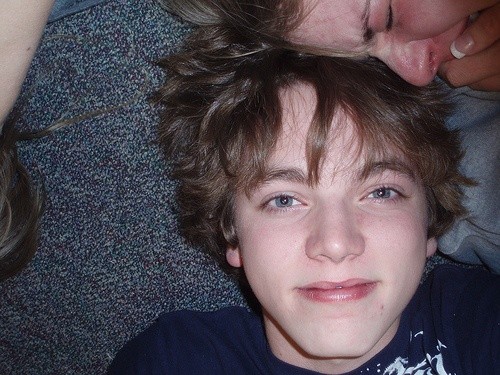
[103,24,500,375]
[159,1,500,93]
